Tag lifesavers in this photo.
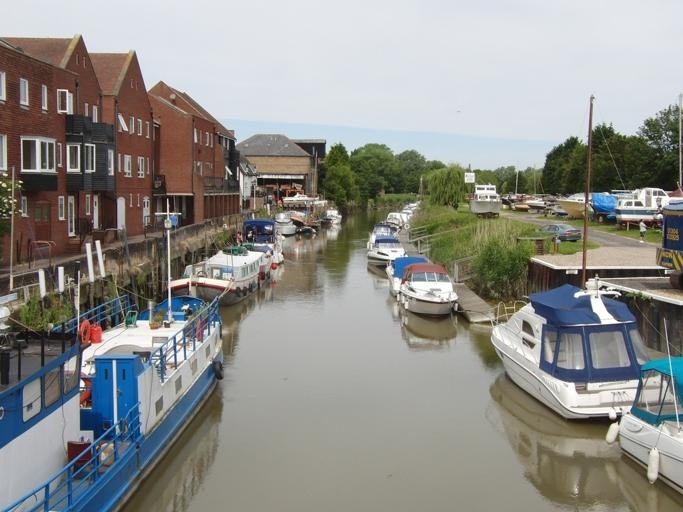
[80,320,90,342]
[79,372,92,402]
[404,223,411,230]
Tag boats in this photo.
[502,187,683,225]
[470,185,500,214]
[366,200,458,315]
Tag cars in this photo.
[540,224,581,242]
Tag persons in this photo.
[170,211,178,230]
[639,219,648,243]
[251,226,257,242]
[237,231,243,247]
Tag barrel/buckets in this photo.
[89,322,103,343]
